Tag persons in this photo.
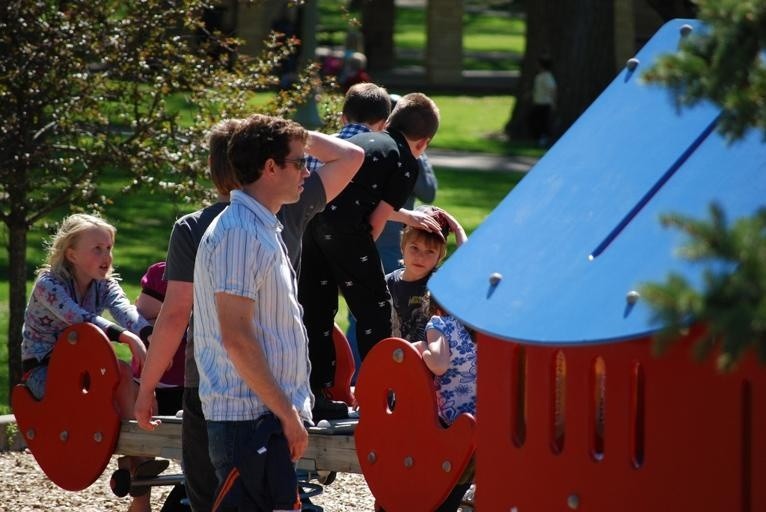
[344,94,436,387]
[190,110,324,512]
[296,91,441,423]
[20,212,176,512]
[383,205,467,341]
[128,261,191,512]
[336,18,366,86]
[299,81,391,169]
[132,114,368,512]
[522,53,560,151]
[406,289,479,428]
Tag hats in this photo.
[412,204,450,243]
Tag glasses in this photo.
[285,158,308,169]
